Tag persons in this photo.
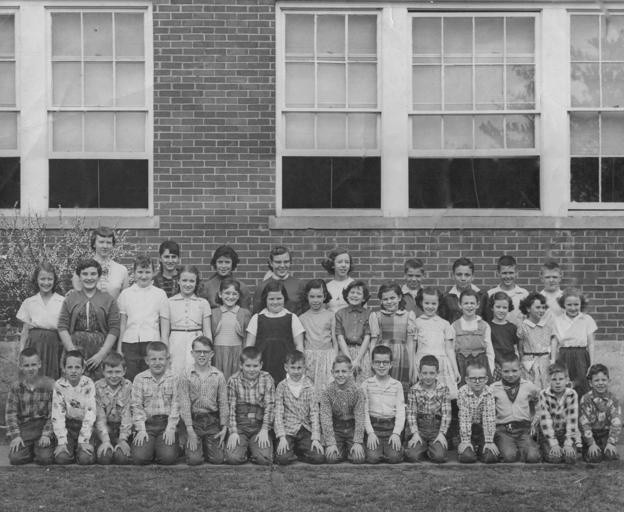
[6,229,623,463]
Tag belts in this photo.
[524,352,548,356]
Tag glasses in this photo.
[195,350,211,355]
[470,377,485,382]
